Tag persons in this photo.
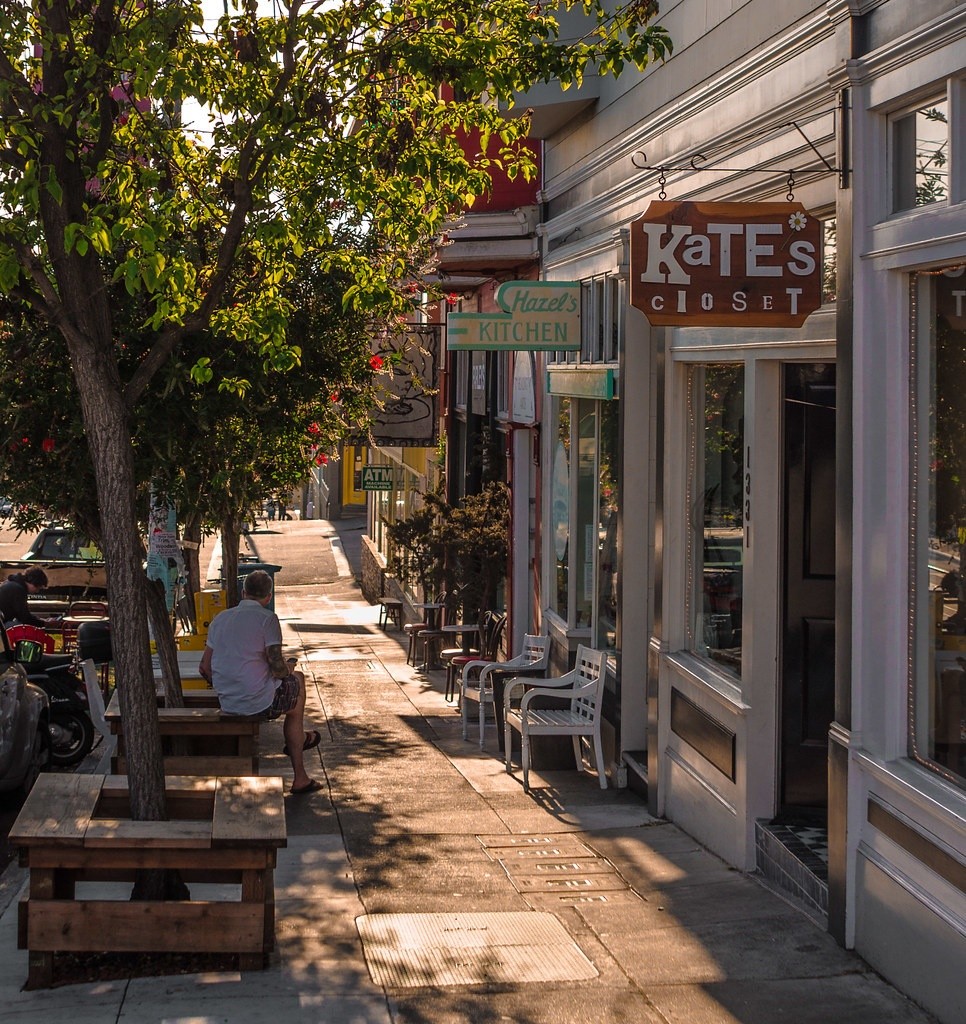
[0,566,61,631]
[199,570,323,793]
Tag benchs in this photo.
[102,688,261,775]
[10,771,287,984]
[378,597,403,631]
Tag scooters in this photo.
[21,650,94,767]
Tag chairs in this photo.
[440,610,493,702]
[452,616,508,712]
[508,644,608,793]
[460,632,552,748]
[417,590,460,674]
[403,591,448,667]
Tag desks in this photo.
[414,603,450,670]
[443,625,488,678]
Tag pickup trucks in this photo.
[0,521,111,617]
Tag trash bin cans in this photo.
[238,563,283,612]
[521,680,583,771]
[491,670,544,752]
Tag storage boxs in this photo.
[178,589,227,650]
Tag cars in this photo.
[0,615,54,807]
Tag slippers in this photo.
[289,779,324,794]
[283,730,321,756]
